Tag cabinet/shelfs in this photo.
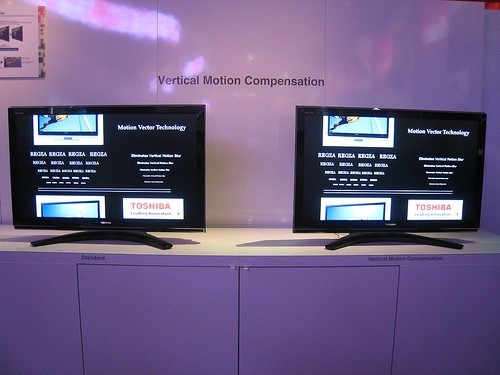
[0,252,500,374]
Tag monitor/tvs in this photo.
[0,26,26,68]
[293,105,487,235]
[7,105,209,234]
